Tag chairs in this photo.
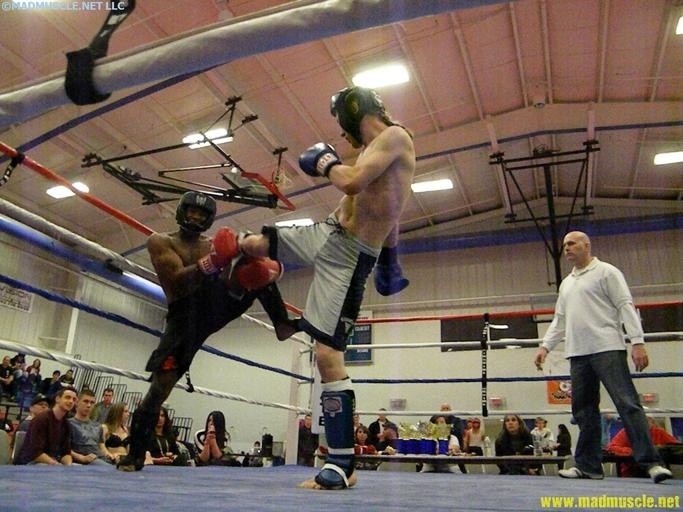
[0,429,15,466]
[12,429,26,458]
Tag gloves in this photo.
[298,142,343,177]
[238,255,284,289]
[373,246,410,297]
[197,226,239,278]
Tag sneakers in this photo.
[647,464,673,484]
[557,463,605,480]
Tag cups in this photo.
[355,446,360,455]
[362,446,368,454]
[166,453,172,460]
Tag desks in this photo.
[313,451,567,475]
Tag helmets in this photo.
[176,189,217,232]
[328,85,385,142]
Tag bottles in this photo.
[532,427,543,456]
[483,436,492,458]
[396,437,449,455]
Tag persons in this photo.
[531,231,671,483]
[350,404,485,478]
[0,350,275,467]
[116,190,306,473]
[298,415,318,466]
[495,413,679,477]
[228,86,415,486]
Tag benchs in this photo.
[0,384,36,423]
